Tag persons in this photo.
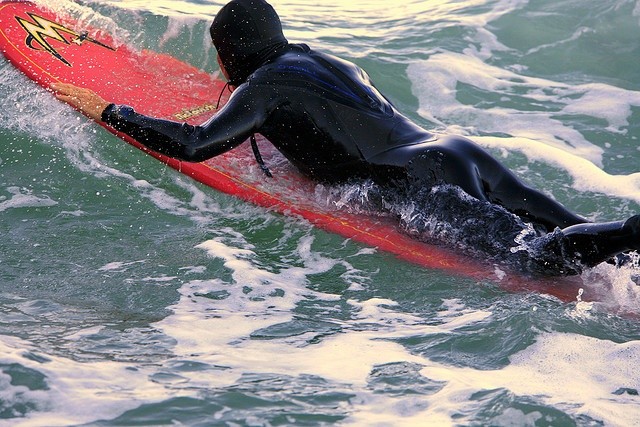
[48,1,640,281]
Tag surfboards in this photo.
[0,1,636,319]
